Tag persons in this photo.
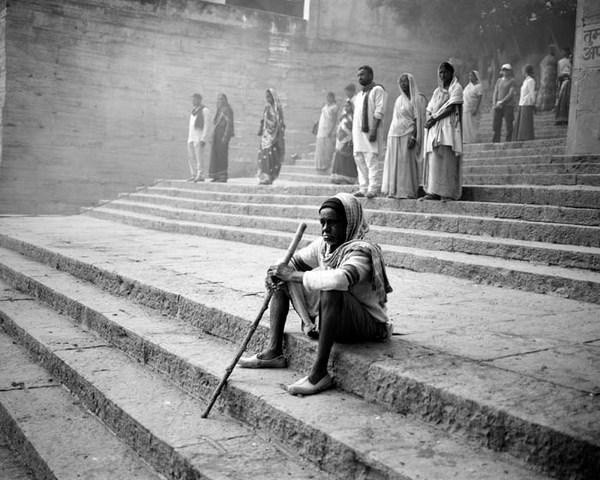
[489,63,516,143]
[353,65,389,199]
[512,62,538,141]
[338,82,358,116]
[256,88,286,185]
[460,68,486,144]
[313,87,342,170]
[234,192,394,396]
[331,99,359,184]
[535,42,560,113]
[416,60,464,202]
[381,71,428,200]
[208,93,234,183]
[187,93,211,182]
[551,45,574,125]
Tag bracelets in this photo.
[411,135,417,141]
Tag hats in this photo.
[502,64,512,69]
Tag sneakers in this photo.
[287,376,330,394]
[353,191,377,198]
[239,354,287,369]
